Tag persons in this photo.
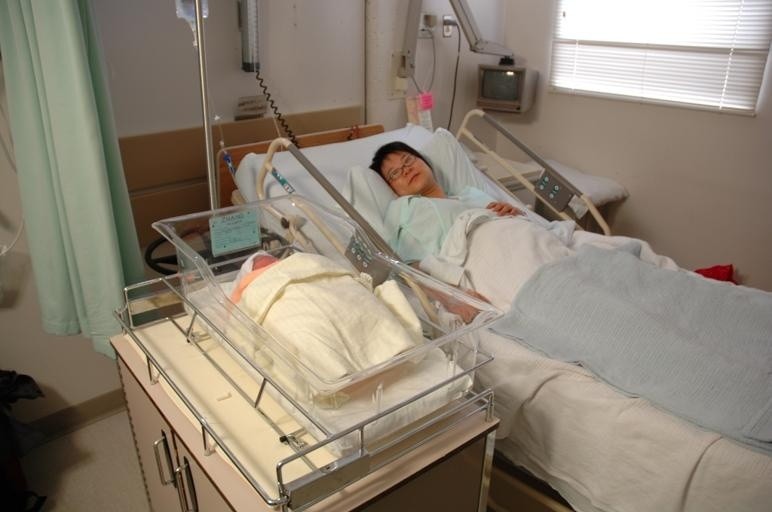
[238,249,279,274]
[368,140,529,323]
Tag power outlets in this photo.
[417,13,458,44]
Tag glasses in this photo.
[387,153,416,182]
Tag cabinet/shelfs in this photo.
[107,334,500,509]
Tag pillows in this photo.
[335,128,489,247]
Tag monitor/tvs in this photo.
[477,63,539,113]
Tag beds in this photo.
[214,119,771,508]
